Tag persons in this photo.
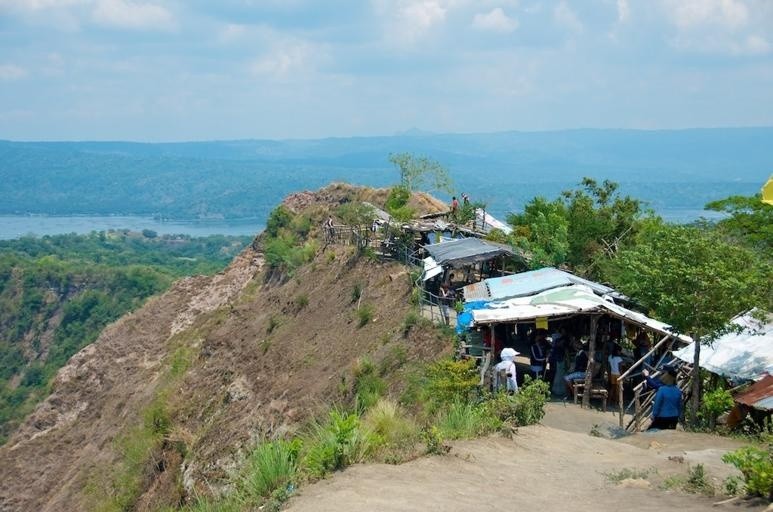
[481,321,683,430]
[451,196,458,210]
[323,214,334,239]
[448,273,453,285]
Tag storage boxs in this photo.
[573,382,608,413]
